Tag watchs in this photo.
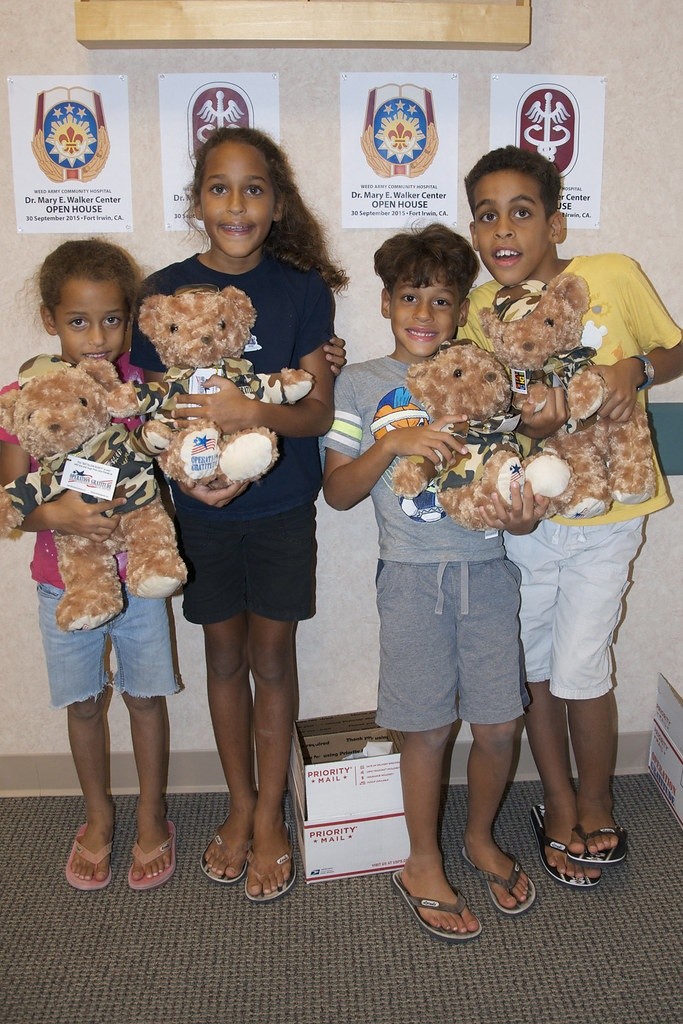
[630,355,655,391]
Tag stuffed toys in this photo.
[474,273,657,522]
[1,354,189,632]
[392,343,574,532]
[104,286,314,487]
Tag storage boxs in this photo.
[287,708,411,884]
[647,673,683,831]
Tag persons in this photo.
[447,147,683,889]
[323,219,551,940]
[126,123,351,902]
[0,240,346,890]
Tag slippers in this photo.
[567,824,627,868]
[461,846,537,917]
[391,869,483,944]
[530,804,602,891]
[244,822,297,903]
[128,821,176,890]
[66,822,113,891]
[199,813,253,886]
